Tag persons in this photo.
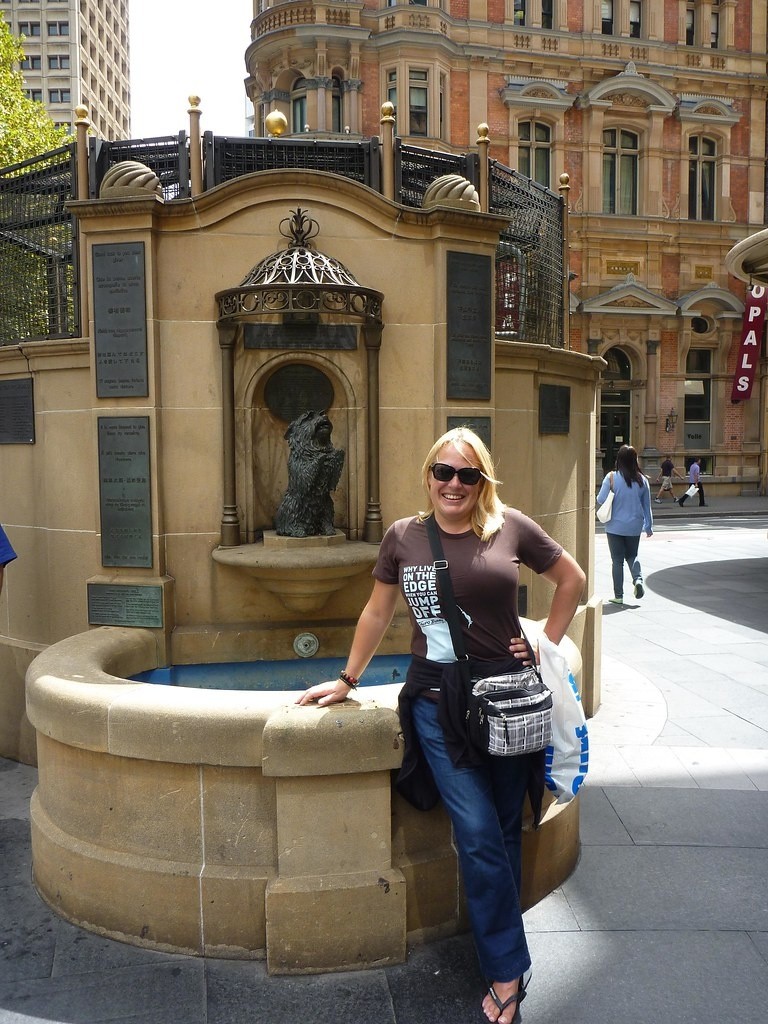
[679,457,707,507]
[596,445,654,604]
[294,426,587,1024]
[654,454,684,504]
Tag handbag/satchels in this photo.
[596,471,615,524]
[685,484,699,498]
[467,665,554,757]
[532,633,589,805]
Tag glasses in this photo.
[430,463,484,485]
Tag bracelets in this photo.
[339,670,360,691]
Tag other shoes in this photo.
[633,580,645,599]
[699,503,708,507]
[678,499,683,507]
[608,598,623,604]
[673,497,681,502]
[654,498,662,503]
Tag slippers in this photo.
[481,974,523,1024]
